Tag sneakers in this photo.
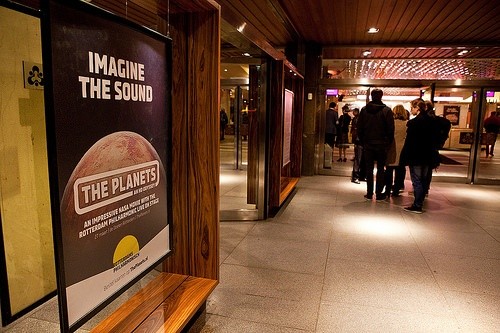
[404,205,423,214]
[363,194,372,201]
[376,193,387,203]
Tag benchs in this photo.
[88,271,219,333]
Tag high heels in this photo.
[337,157,347,162]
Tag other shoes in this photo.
[391,183,404,191]
[384,189,400,198]
[351,174,367,184]
[408,188,429,198]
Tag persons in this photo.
[219,106,229,140]
[397,98,440,214]
[335,106,352,162]
[408,100,440,196]
[484,111,500,156]
[350,108,362,164]
[325,101,339,161]
[240,111,249,141]
[355,87,395,202]
[389,108,413,194]
[381,104,407,199]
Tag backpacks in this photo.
[425,115,451,150]
